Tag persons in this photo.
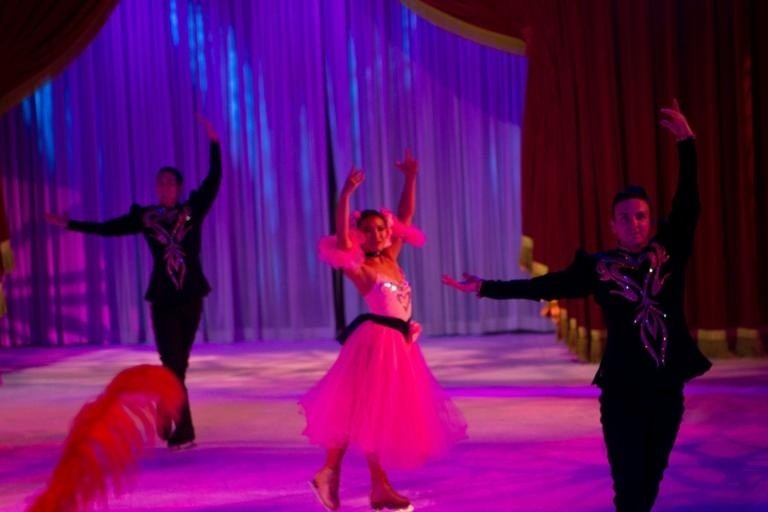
[43,112,222,447]
[297,146,469,512]
[441,96,714,512]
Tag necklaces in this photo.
[365,252,381,256]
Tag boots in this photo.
[371,477,411,508]
[312,466,340,509]
[155,404,173,440]
[167,427,195,447]
